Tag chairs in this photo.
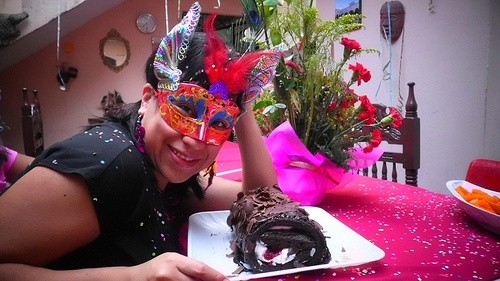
[21,87,44,157]
[356,82,420,187]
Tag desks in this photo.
[171,139,500,281]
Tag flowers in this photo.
[236,1,404,203]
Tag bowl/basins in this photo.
[446,179,500,229]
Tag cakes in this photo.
[227,184,331,274]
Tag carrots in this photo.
[456,186,500,215]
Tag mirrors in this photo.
[100,29,131,72]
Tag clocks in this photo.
[136,13,158,34]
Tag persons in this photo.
[0,31,278,281]
[0,145,36,193]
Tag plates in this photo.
[187,205,386,281]
